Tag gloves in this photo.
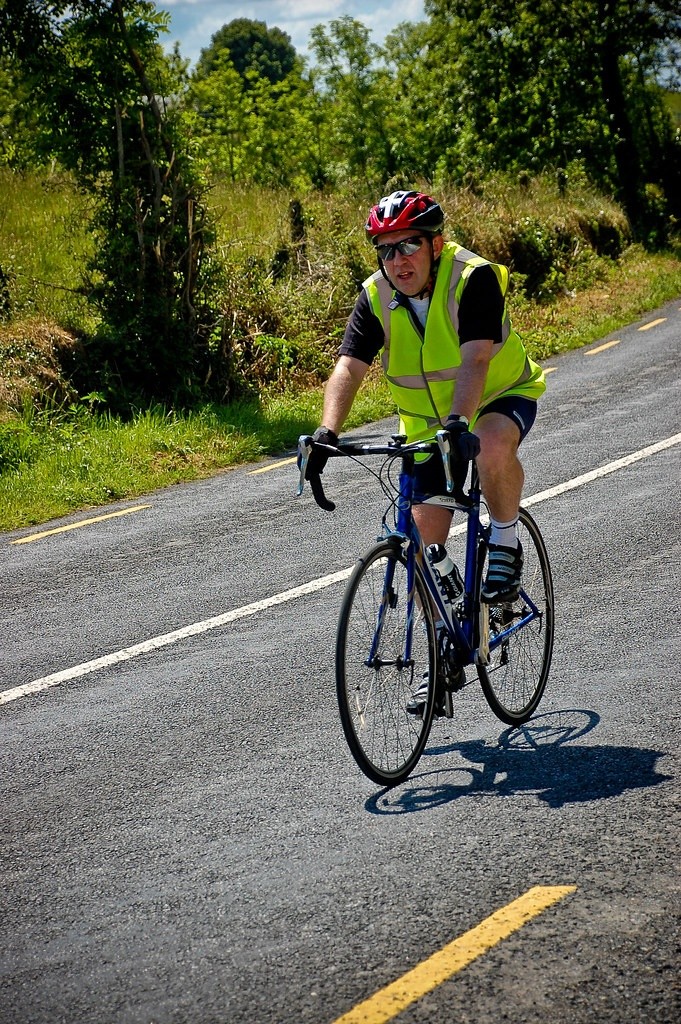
[439,414,480,468]
[296,426,339,481]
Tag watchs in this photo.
[446,414,469,427]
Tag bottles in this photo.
[425,544,465,603]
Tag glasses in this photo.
[375,234,424,261]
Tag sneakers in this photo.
[479,537,525,604]
[405,663,445,714]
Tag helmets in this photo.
[364,189,444,249]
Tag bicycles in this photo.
[297,434,554,784]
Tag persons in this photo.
[296,190,546,714]
